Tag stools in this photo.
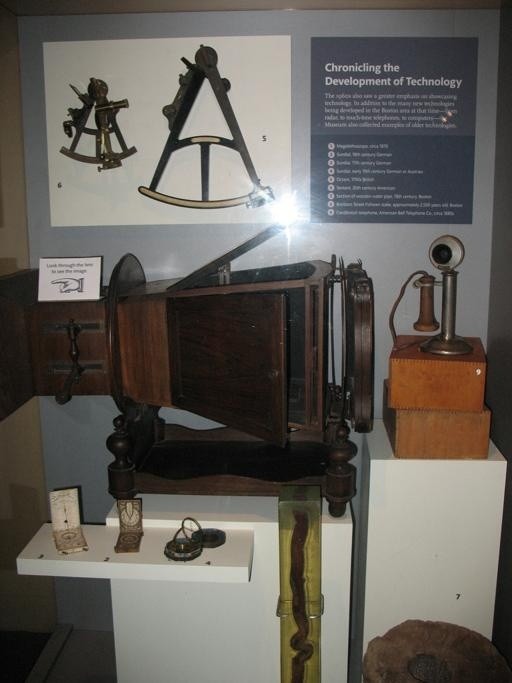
[363,619,512,683]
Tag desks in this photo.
[359,419,508,660]
[106,497,353,683]
